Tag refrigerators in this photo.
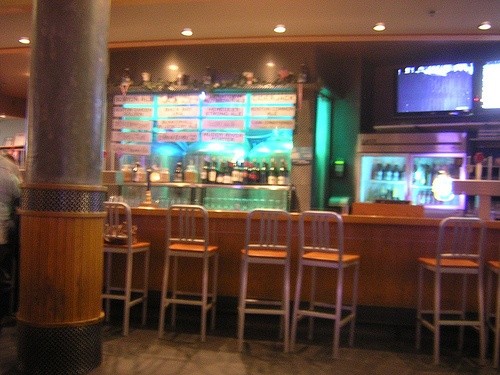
[354,132,468,212]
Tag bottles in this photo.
[369,162,460,206]
[122,156,288,186]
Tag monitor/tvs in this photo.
[392,61,475,122]
[475,58,500,119]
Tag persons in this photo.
[0,151,24,328]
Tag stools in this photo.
[103,202,359,356]
[414,215,500,369]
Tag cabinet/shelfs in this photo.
[104,85,304,218]
